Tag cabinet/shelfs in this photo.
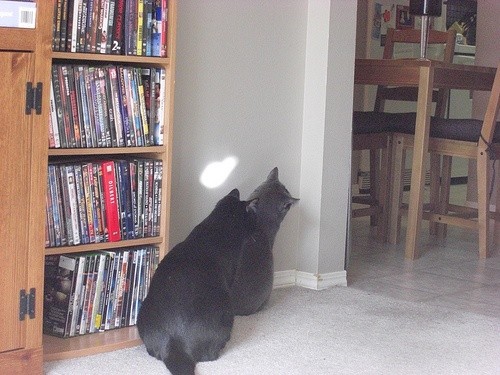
[42,0,179,360]
[0,0,56,375]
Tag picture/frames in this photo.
[395,4,415,30]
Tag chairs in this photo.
[352,27,457,244]
[385,63,500,261]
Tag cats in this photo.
[136,166,300,375]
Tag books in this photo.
[45,161,165,249]
[43,244,160,340]
[48,63,166,148]
[52,1,168,59]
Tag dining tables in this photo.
[346,59,499,261]
